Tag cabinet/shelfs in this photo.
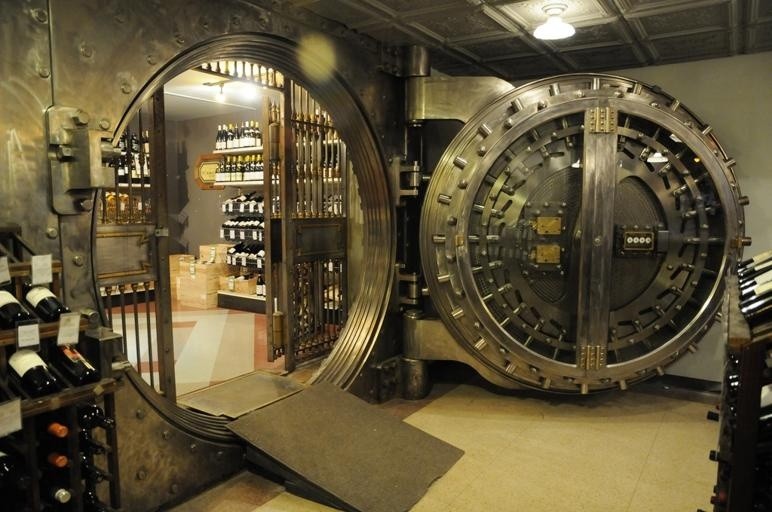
[0,219,124,510]
[709,261,771,510]
[211,143,343,324]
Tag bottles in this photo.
[693,246,771,512]
[0,286,33,330]
[53,339,101,389]
[0,399,117,511]
[4,343,62,399]
[22,276,71,323]
[94,129,153,226]
[216,112,341,302]
